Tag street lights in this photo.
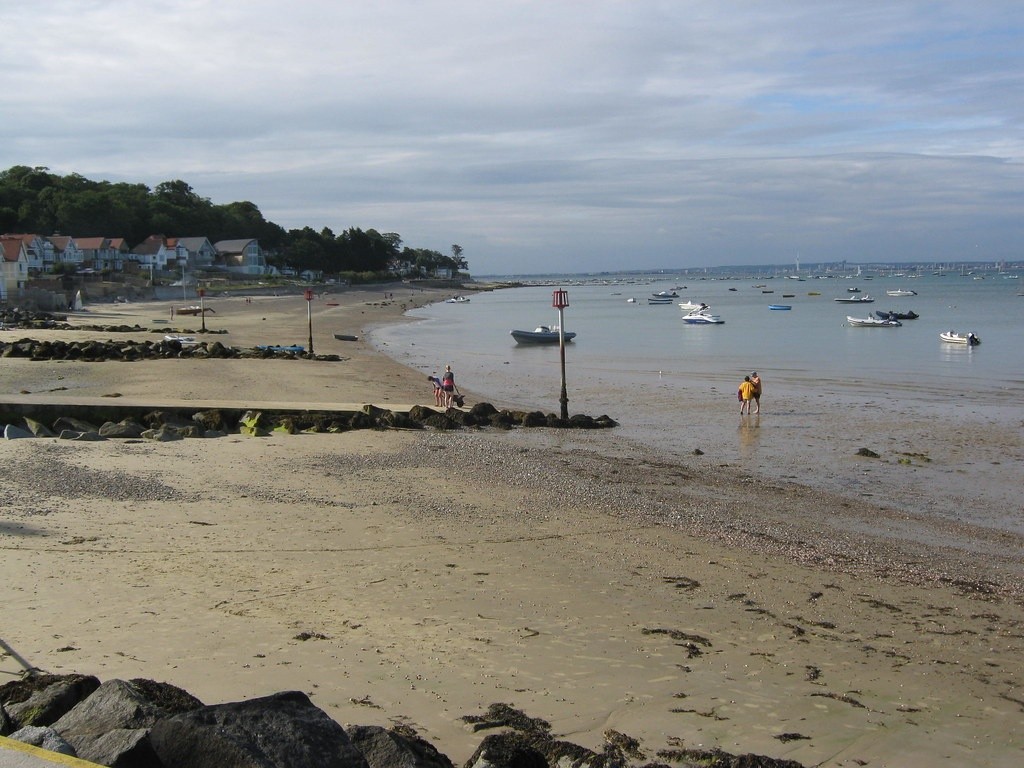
[196,286,206,331]
[552,287,570,419]
[304,289,314,353]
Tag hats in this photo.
[751,371,756,375]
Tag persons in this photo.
[867,312,874,319]
[888,311,897,321]
[750,371,761,414]
[738,376,759,414]
[443,365,455,409]
[428,376,443,407]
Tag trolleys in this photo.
[434,389,465,408]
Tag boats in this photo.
[334,334,359,342]
[846,316,902,327]
[966,332,980,345]
[522,272,1019,297]
[458,299,470,303]
[682,306,725,324]
[940,332,969,345]
[679,301,699,310]
[510,325,576,344]
[648,298,674,305]
[834,296,875,303]
[446,299,457,303]
[875,310,919,320]
[627,298,637,303]
[326,303,340,306]
